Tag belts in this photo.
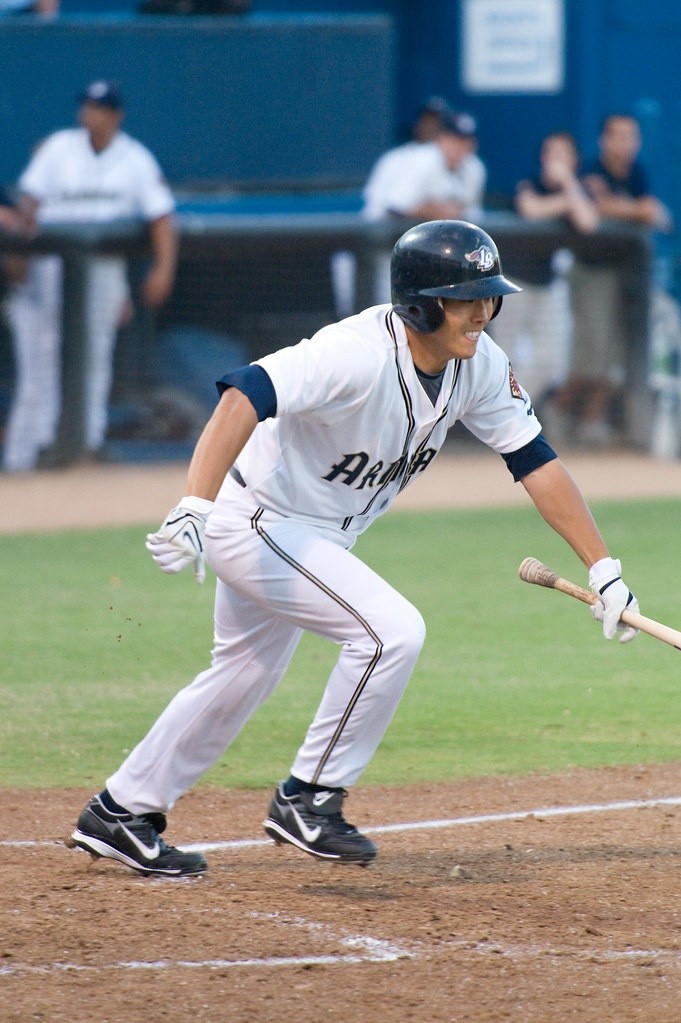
[228,465,246,488]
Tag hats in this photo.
[80,81,123,110]
[443,112,482,143]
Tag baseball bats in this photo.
[517,556,681,650]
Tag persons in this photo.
[1,84,676,463]
[64,221,641,879]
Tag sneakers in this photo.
[64,794,208,879]
[262,781,376,866]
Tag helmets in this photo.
[390,220,524,336]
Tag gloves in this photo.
[145,495,215,585]
[589,557,641,643]
